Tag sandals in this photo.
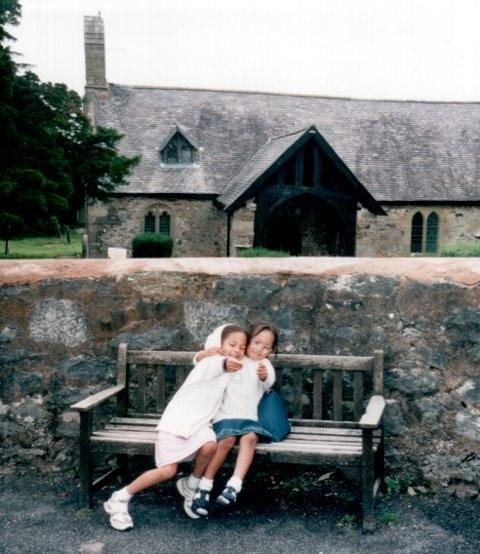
[191,488,210,517]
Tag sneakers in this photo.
[216,487,238,505]
[104,497,134,531]
[176,476,200,519]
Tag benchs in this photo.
[65,341,388,534]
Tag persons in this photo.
[191,320,278,516]
[102,324,247,531]
